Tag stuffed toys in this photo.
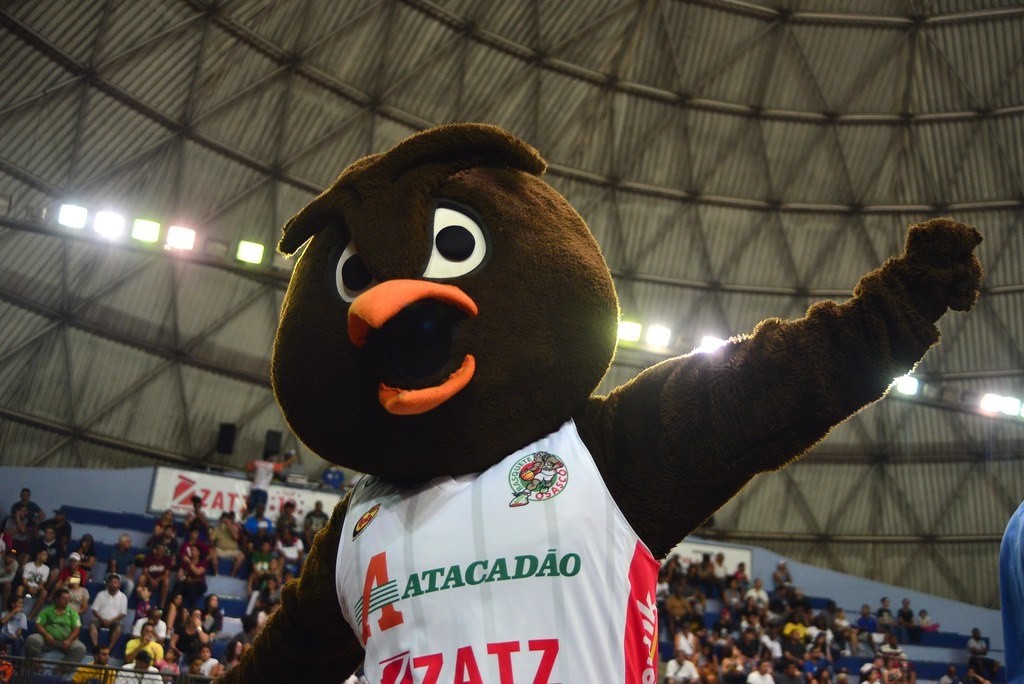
[209,122,985,684]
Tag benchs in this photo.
[5,504,304,684]
[658,569,1005,684]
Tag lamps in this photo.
[27,204,87,230]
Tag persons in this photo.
[759,626,782,662]
[746,660,775,684]
[802,646,831,684]
[664,649,700,684]
[125,622,164,666]
[242,451,299,521]
[722,574,746,615]
[131,607,167,647]
[71,645,116,684]
[89,574,128,650]
[115,649,164,684]
[0,463,1024,684]
[75,534,95,583]
[173,543,208,609]
[674,621,701,666]
[10,488,45,538]
[209,512,245,578]
[24,589,87,679]
[104,534,137,599]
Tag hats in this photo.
[146,607,162,618]
[69,552,81,561]
[779,559,788,565]
[52,507,69,516]
